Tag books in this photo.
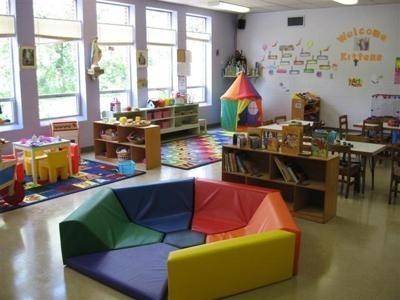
[222,121,330,185]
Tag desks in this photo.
[12,137,72,185]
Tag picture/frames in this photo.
[19,47,37,70]
[136,49,148,68]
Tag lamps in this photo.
[207,1,250,14]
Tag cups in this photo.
[135,116,141,121]
[119,116,127,125]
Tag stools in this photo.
[199,119,208,135]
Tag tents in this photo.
[219,72,263,134]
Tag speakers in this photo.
[238,19,246,29]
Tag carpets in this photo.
[161,127,234,171]
[1,159,147,214]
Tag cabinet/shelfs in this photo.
[114,101,201,138]
[220,145,339,225]
[92,121,162,171]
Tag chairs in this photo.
[257,96,400,207]
[1,143,80,184]
